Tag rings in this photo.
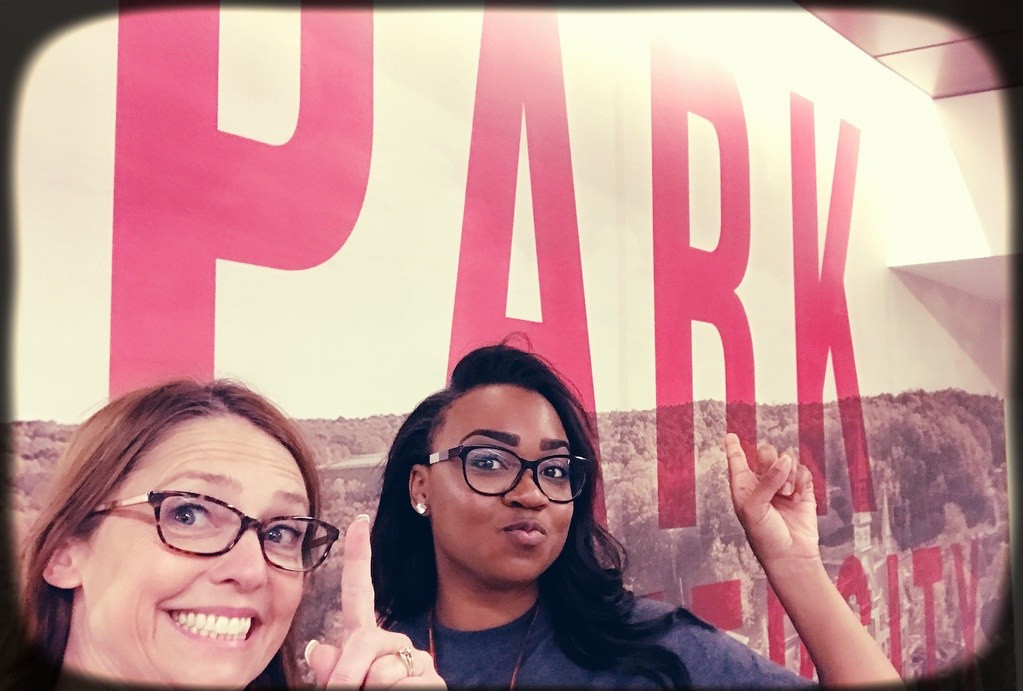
[396,646,415,678]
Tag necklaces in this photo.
[428,597,541,691]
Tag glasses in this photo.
[425,444,594,504]
[90,490,339,573]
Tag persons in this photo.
[370,332,902,691]
[15,376,447,691]
